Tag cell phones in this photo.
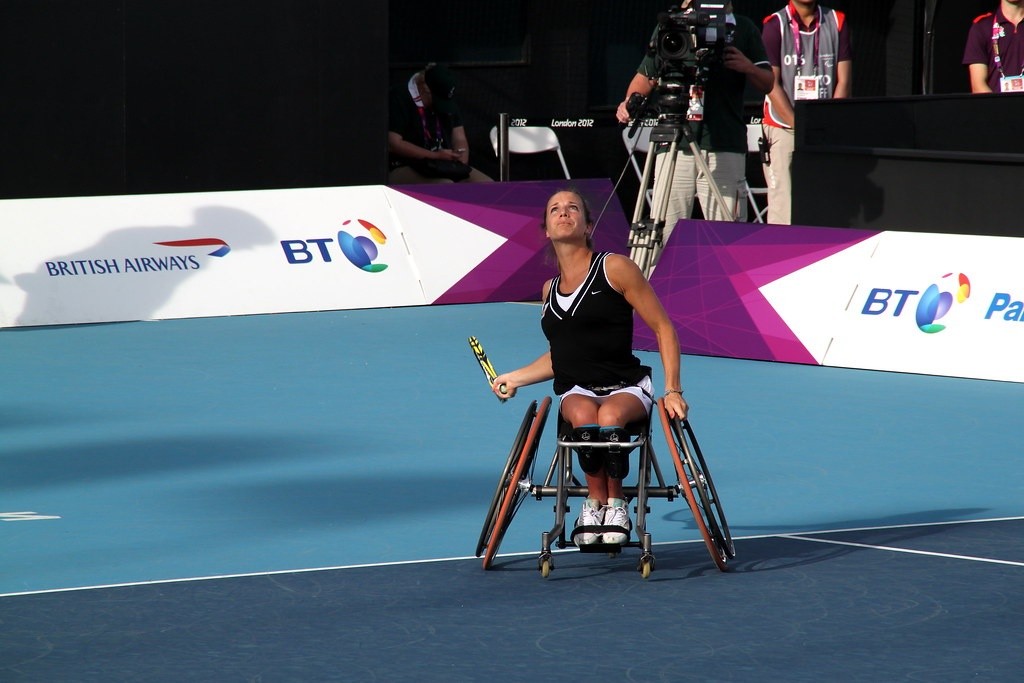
[453,149,466,154]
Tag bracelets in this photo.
[661,389,684,395]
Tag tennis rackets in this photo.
[468,336,509,405]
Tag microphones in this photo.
[658,12,673,27]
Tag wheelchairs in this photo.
[474,397,736,579]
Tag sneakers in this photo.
[602,496,631,544]
[574,494,607,545]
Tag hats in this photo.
[425,61,464,110]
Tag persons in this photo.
[962,0,1024,94]
[797,82,804,90]
[761,0,855,226]
[1005,83,1009,90]
[387,62,494,185]
[616,0,776,282]
[494,189,689,546]
